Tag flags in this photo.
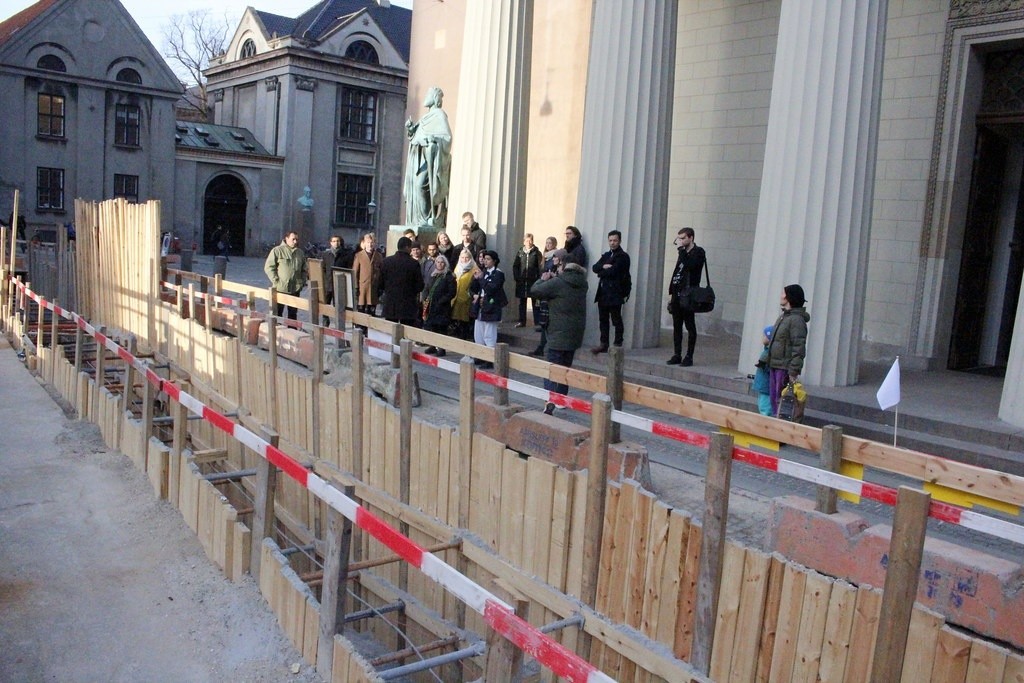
[875,359,900,410]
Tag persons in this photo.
[666,227,706,367]
[530,254,589,416]
[264,230,308,330]
[513,227,586,332]
[213,224,232,263]
[298,186,314,207]
[404,88,452,227]
[752,284,810,447]
[322,212,505,369]
[591,230,631,355]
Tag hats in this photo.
[483,250,500,263]
[764,327,774,340]
[554,249,567,260]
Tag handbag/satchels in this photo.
[422,294,432,321]
[683,286,715,313]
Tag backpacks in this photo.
[777,382,807,423]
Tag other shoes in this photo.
[436,351,445,356]
[425,346,436,353]
[613,337,623,347]
[555,403,566,409]
[515,322,526,327]
[528,351,544,359]
[479,362,493,369]
[667,355,682,365]
[542,402,555,415]
[474,359,484,365]
[679,356,693,366]
[591,343,609,354]
